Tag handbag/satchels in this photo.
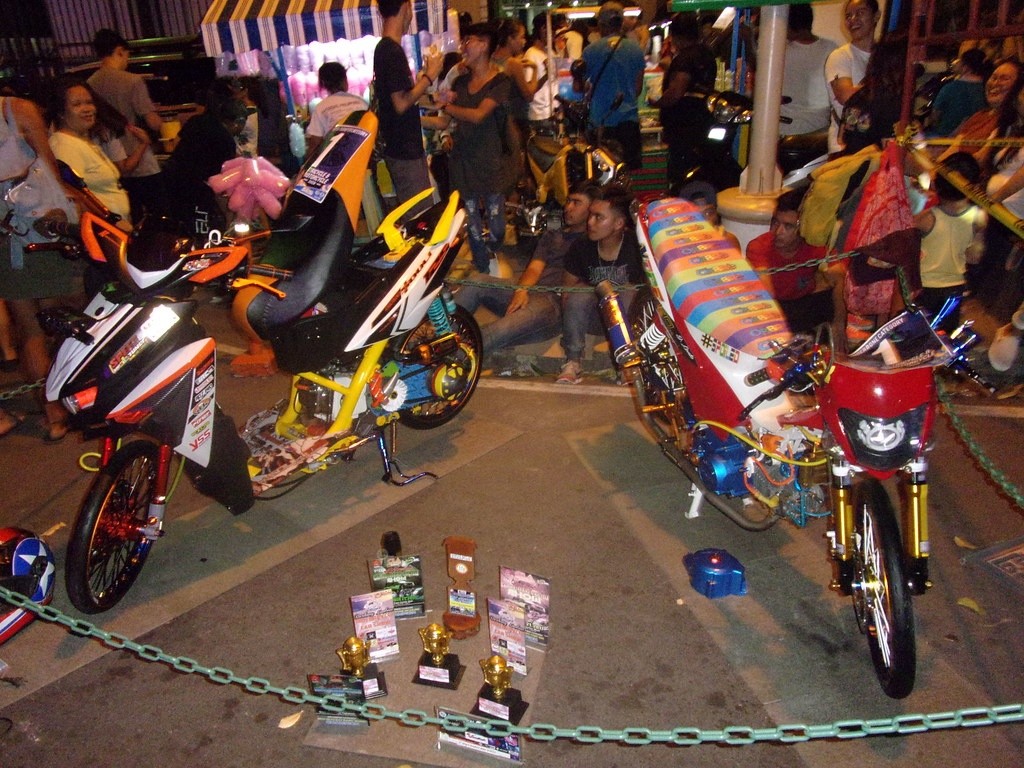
[573,100,589,132]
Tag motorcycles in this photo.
[592,190,984,700]
[43,109,485,614]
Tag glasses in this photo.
[461,36,487,45]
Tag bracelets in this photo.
[422,74,433,86]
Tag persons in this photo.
[0,0,1024,443]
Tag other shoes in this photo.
[46,410,67,442]
[0,409,25,437]
[555,360,581,385]
[488,249,499,279]
[617,365,633,386]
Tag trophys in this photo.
[335,637,388,703]
[469,655,529,725]
[411,621,467,690]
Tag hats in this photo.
[597,2,624,30]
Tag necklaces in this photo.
[599,242,620,279]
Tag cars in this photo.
[65,34,198,107]
